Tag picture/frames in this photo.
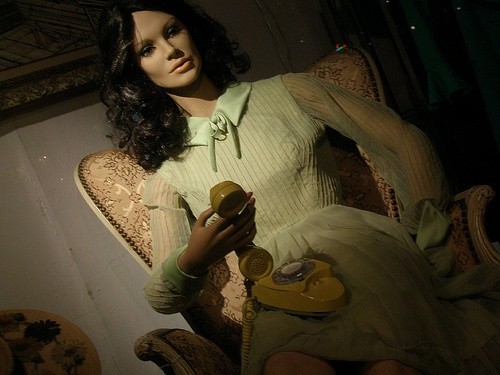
[0,0,122,142]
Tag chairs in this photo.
[74,44,500,375]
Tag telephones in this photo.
[209,180,348,317]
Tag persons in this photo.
[96,0,500,374]
